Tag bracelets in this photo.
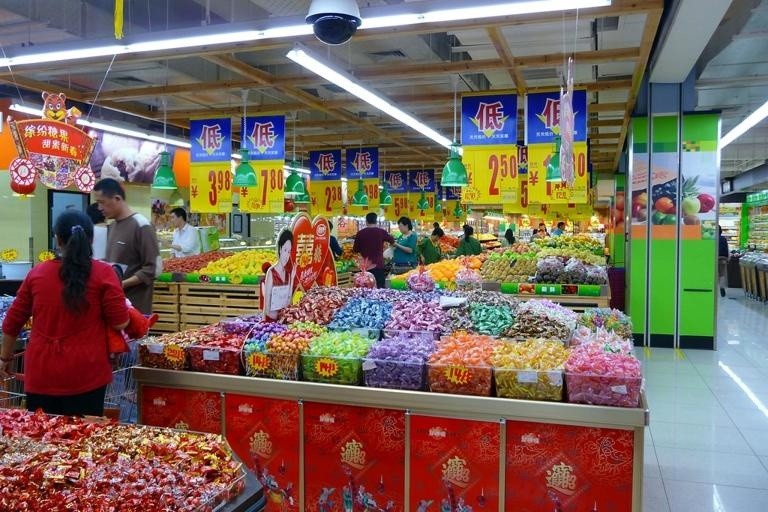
[0,356,14,362]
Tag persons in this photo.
[87,201,112,260]
[393,216,418,275]
[167,207,202,257]
[0,209,130,420]
[353,212,394,288]
[457,224,482,256]
[262,229,298,323]
[718,225,730,298]
[501,228,518,247]
[327,220,345,263]
[418,222,444,266]
[92,178,160,318]
[529,222,565,243]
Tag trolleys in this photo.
[0,314,158,421]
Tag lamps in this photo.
[231,89,257,188]
[0,0,614,68]
[284,42,464,158]
[7,99,385,191]
[417,163,429,210]
[169,188,184,208]
[151,96,178,190]
[435,169,442,214]
[351,141,369,207]
[12,191,37,198]
[545,66,577,183]
[293,142,311,204]
[482,205,507,221]
[440,73,469,186]
[284,109,305,195]
[466,203,473,215]
[454,187,463,217]
[379,147,392,207]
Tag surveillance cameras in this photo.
[303,0,363,47]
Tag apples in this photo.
[697,192,715,212]
[683,197,701,214]
[656,196,674,212]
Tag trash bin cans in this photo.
[608,266,626,311]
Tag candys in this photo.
[300,329,377,387]
[277,286,514,331]
[138,329,193,371]
[500,298,576,340]
[570,306,634,341]
[189,320,255,376]
[492,337,568,400]
[427,331,495,397]
[0,408,244,512]
[363,331,435,391]
[564,341,642,407]
[242,320,320,383]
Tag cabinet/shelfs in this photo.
[724,250,751,288]
[392,232,612,314]
[1,404,267,512]
[745,200,768,251]
[1,294,31,405]
[132,286,649,512]
[149,248,351,337]
[739,253,768,305]
[719,201,741,248]
